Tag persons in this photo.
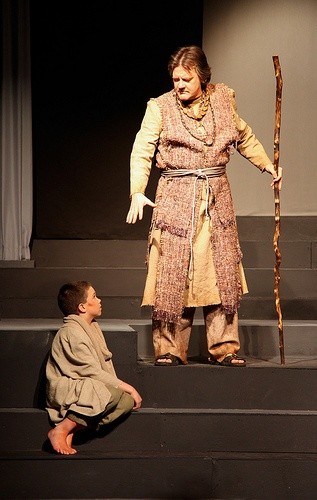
[127,47,282,367]
[45,280,143,456]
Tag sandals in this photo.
[210,353,247,367]
[155,353,182,366]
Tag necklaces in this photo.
[176,93,216,146]
